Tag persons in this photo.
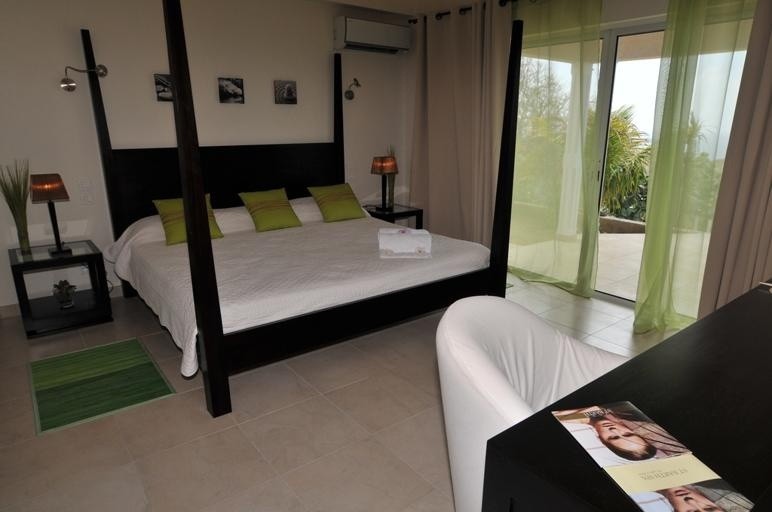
[559,400,656,461]
[657,486,730,512]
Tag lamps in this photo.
[29,171,73,256]
[344,76,361,100]
[369,154,399,211]
[60,63,107,95]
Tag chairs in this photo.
[432,291,628,512]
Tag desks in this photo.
[479,270,770,511]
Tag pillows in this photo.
[304,180,368,225]
[153,194,225,247]
[236,186,303,234]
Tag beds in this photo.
[78,1,535,421]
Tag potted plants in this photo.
[384,142,399,210]
[0,155,35,257]
[52,276,78,309]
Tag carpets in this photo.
[25,335,179,438]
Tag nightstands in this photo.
[359,200,427,230]
[7,238,115,343]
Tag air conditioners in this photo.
[333,14,413,57]
